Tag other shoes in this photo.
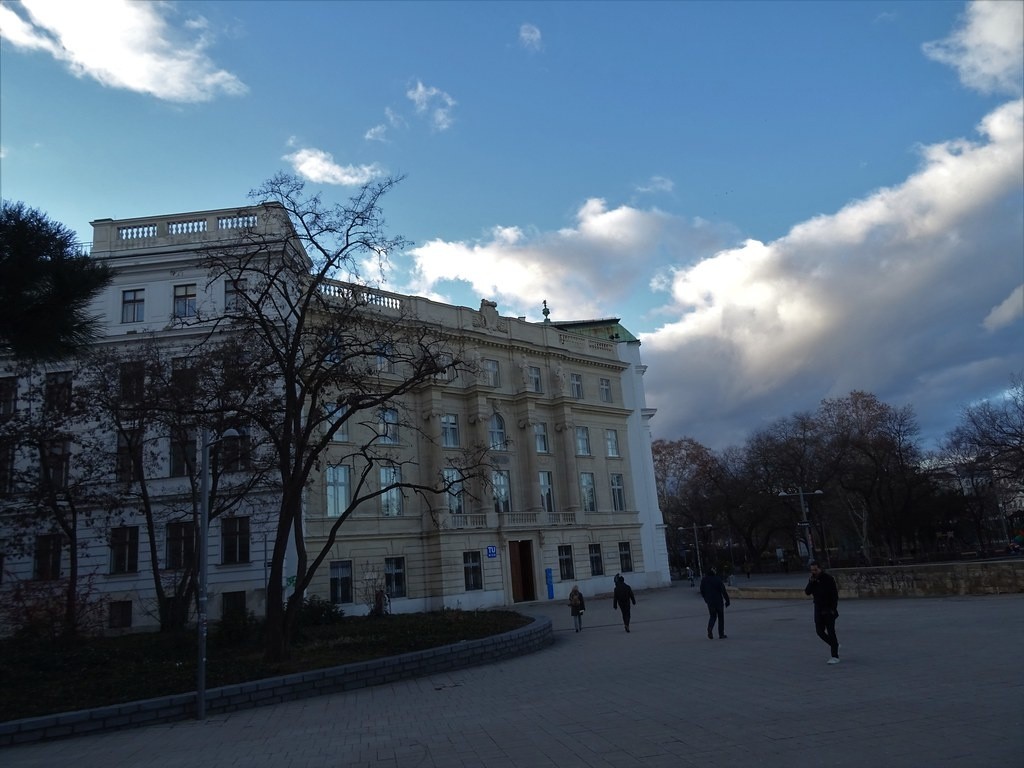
[624,625,630,632]
[719,635,727,639]
[708,628,713,639]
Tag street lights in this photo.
[778,486,824,564]
[193,429,252,718]
[678,522,713,580]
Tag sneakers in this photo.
[826,657,840,664]
[838,644,841,653]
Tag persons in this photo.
[568,584,585,632]
[700,564,730,639]
[804,561,841,664]
[613,572,620,586]
[684,564,730,588]
[613,576,636,633]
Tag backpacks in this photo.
[571,591,581,607]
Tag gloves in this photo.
[725,602,730,608]
